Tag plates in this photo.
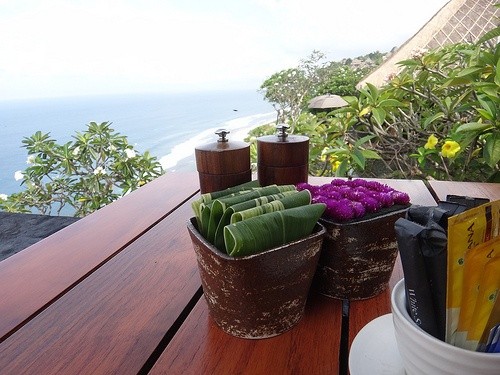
[347,312,395,375]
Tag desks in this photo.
[1,172,499,375]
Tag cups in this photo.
[256,122,309,184]
[317,202,411,301]
[186,214,326,339]
[194,130,252,197]
[390,279,500,374]
